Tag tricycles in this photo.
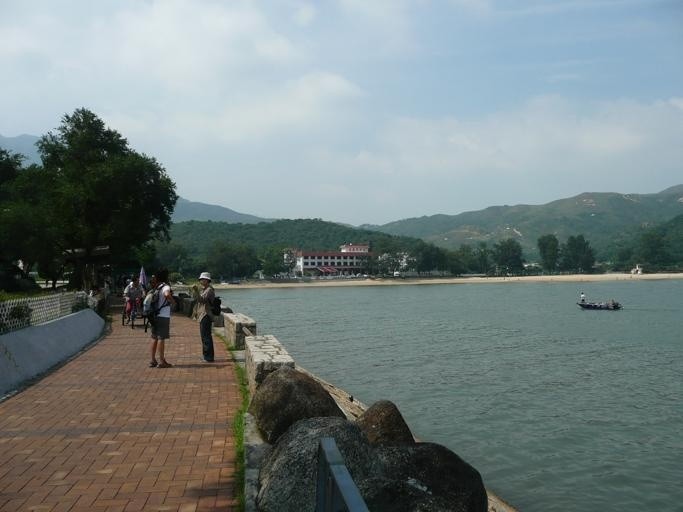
[121,295,146,330]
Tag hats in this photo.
[198,272,212,281]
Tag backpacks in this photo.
[207,287,221,316]
[142,283,172,332]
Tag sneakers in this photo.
[157,361,171,368]
[149,361,157,367]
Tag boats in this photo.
[576,301,623,310]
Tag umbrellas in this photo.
[137,264,148,286]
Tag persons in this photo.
[123,276,145,324]
[147,267,177,369]
[192,270,215,363]
[580,291,585,302]
